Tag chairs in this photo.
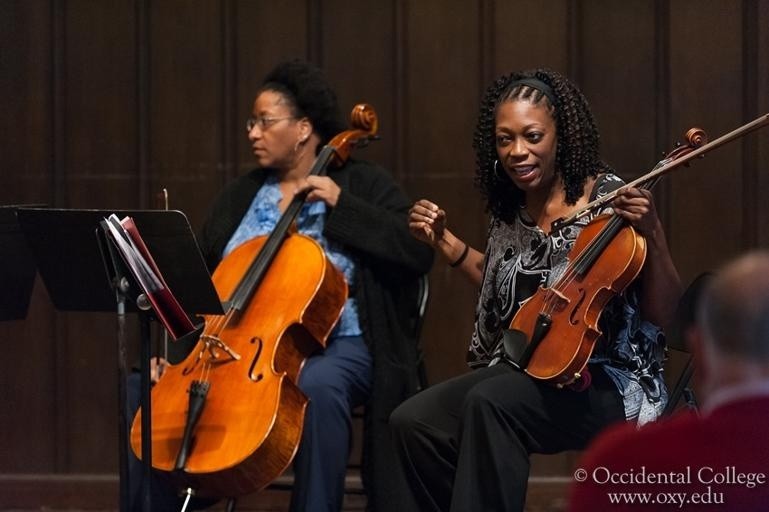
[223,268,431,512]
[550,269,720,474]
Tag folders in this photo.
[103,212,197,342]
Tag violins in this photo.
[504,126,708,389]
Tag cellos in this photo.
[130,103,380,497]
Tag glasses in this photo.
[246,115,306,132]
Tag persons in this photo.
[405,67,686,512]
[123,60,435,512]
[562,248,769,511]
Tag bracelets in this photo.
[447,242,469,268]
